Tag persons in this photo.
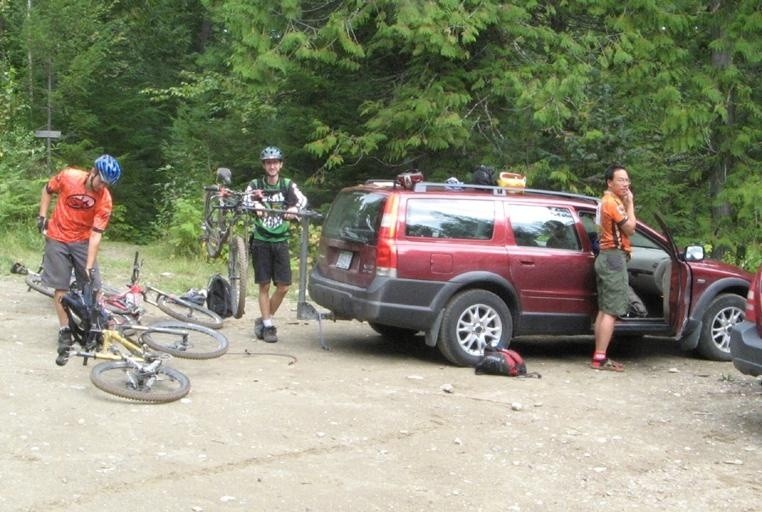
[240,145,309,343]
[588,164,637,372]
[39,153,121,349]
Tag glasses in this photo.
[616,178,632,185]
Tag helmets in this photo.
[470,165,497,192]
[92,153,121,187]
[495,168,529,194]
[257,143,284,163]
[392,167,425,192]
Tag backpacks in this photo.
[472,342,543,383]
[205,274,236,322]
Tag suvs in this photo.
[729,266,762,381]
[305,169,761,368]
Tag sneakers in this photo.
[252,315,280,344]
[590,353,626,374]
[55,325,74,348]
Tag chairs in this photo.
[543,220,579,249]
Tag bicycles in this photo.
[200,180,310,318]
[10,251,231,405]
